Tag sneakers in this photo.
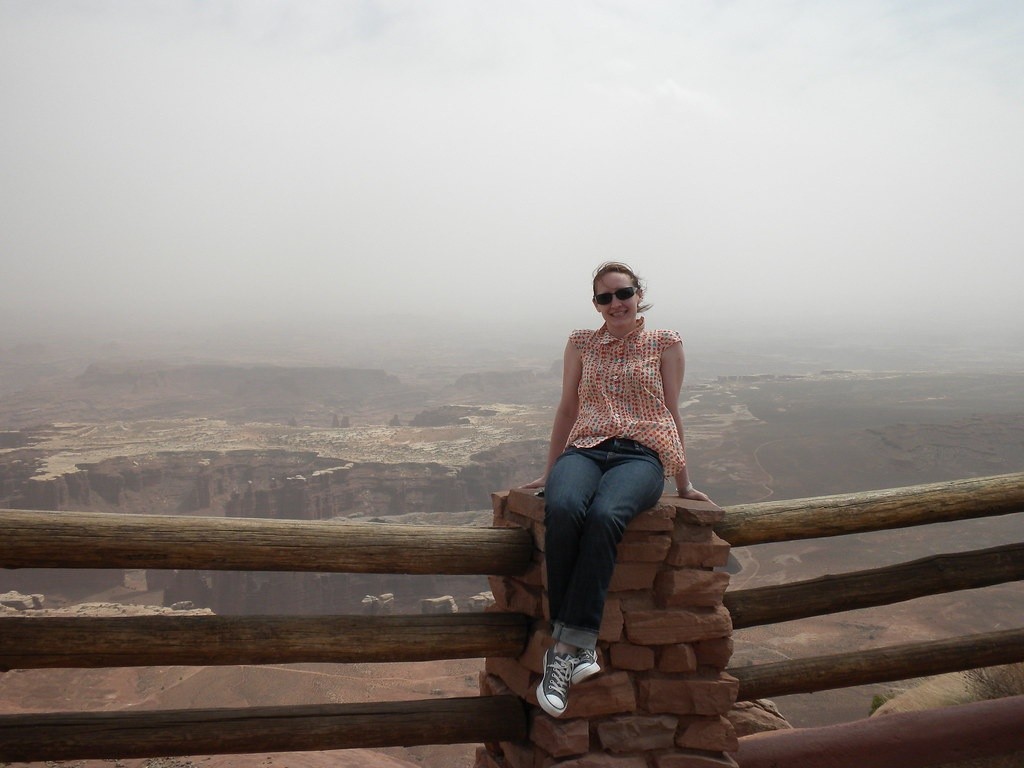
[572,648,600,684]
[536,643,574,719]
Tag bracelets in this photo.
[675,480,693,494]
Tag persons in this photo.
[515,262,724,718]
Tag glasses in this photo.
[593,286,638,305]
